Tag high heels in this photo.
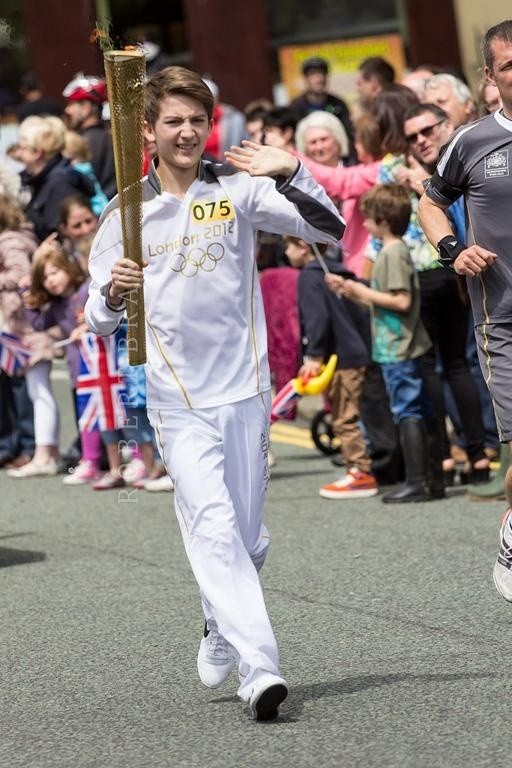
[460,458,489,484]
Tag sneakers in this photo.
[5,442,174,493]
[493,507,512,604]
[321,470,377,497]
[250,678,289,722]
[197,619,234,689]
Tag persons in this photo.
[0,18,510,725]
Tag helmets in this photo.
[62,72,109,104]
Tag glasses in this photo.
[404,119,445,143]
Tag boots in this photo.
[383,417,447,502]
